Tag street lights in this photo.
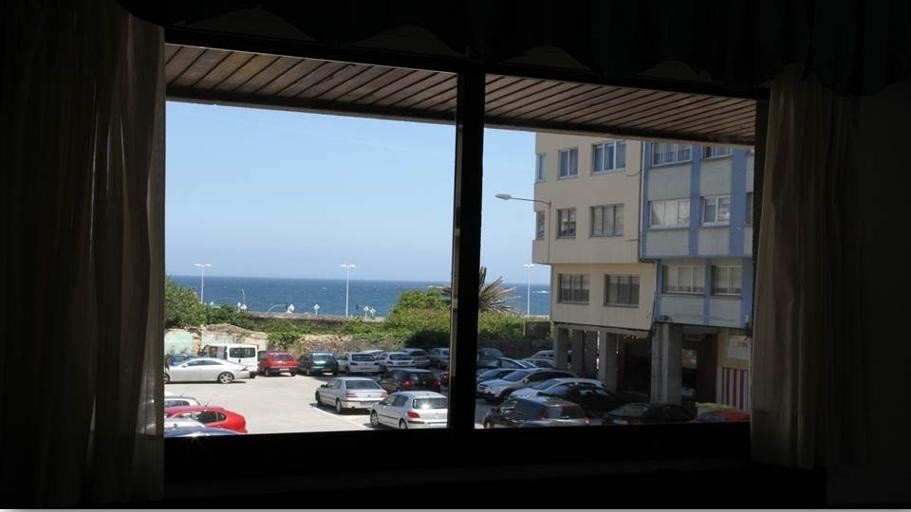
[495,192,550,320]
[194,263,212,303]
[340,264,357,317]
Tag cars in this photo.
[297,348,449,431]
[164,396,247,438]
[163,344,297,384]
[475,348,697,429]
